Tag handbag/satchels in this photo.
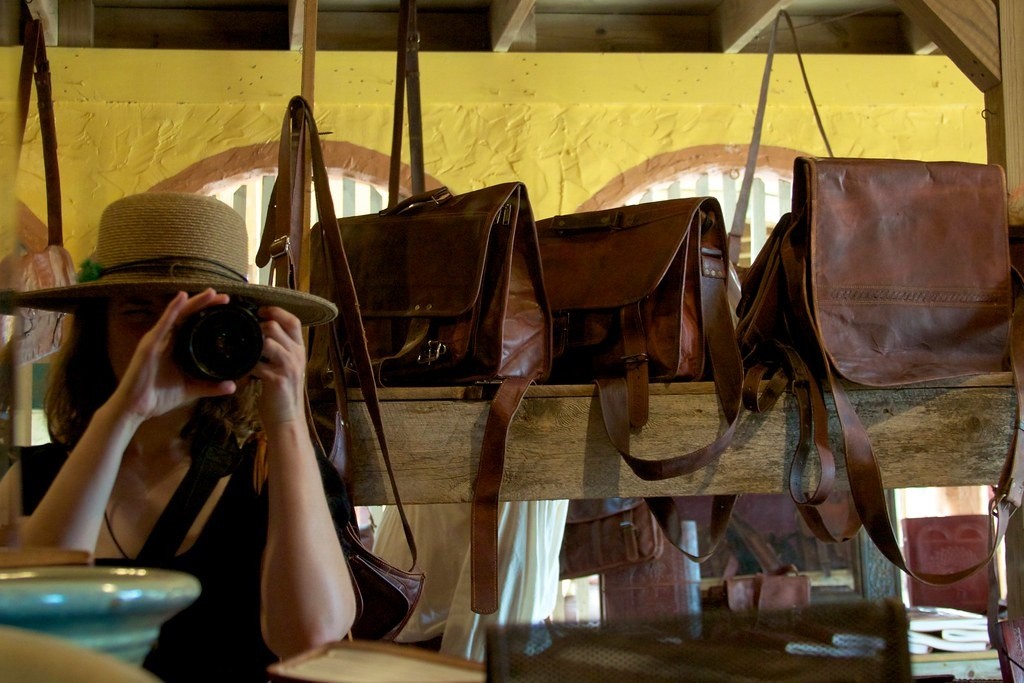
[0,245,77,365]
[534,197,743,481]
[310,183,553,616]
[338,522,425,642]
[700,564,811,612]
[736,156,1024,682]
[558,496,663,579]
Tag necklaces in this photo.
[103,507,136,560]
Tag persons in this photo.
[0,189,358,683]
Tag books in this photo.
[906,604,990,655]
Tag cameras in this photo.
[170,298,268,383]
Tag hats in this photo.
[16,193,338,327]
